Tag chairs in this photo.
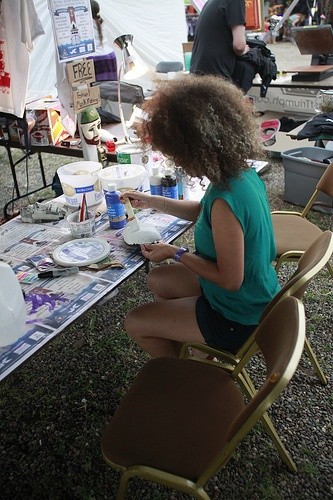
[180,230,328,435]
[146,60,185,97]
[271,162,333,279]
[101,296,305,500]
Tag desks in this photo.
[249,74,333,116]
[286,118,333,165]
[0,121,132,220]
[0,151,271,382]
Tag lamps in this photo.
[114,33,150,143]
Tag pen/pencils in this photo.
[75,192,93,222]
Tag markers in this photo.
[38,265,80,279]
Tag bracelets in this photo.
[175,248,189,261]
[164,197,167,214]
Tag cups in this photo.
[68,211,95,238]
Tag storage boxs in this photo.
[29,98,70,146]
[281,147,333,215]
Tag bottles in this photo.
[105,183,126,229]
[149,166,187,200]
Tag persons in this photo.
[190,0,251,82]
[268,2,312,43]
[90,0,108,54]
[121,76,283,360]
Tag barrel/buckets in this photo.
[57,161,103,208]
[98,164,146,194]
[116,144,153,192]
